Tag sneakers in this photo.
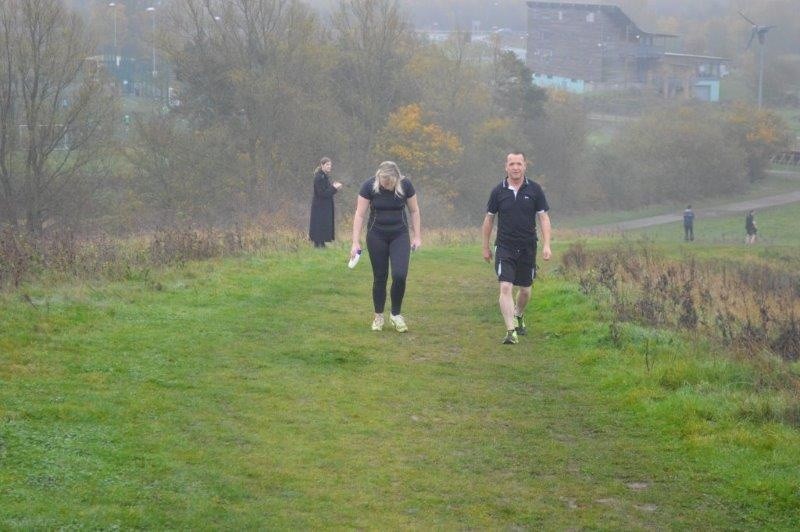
[513,306,527,335]
[388,312,408,333]
[372,313,385,331]
[503,329,518,344]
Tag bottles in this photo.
[349,250,361,268]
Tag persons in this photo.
[309,157,342,247]
[683,205,696,242]
[745,210,758,244]
[348,161,421,332]
[483,150,552,345]
